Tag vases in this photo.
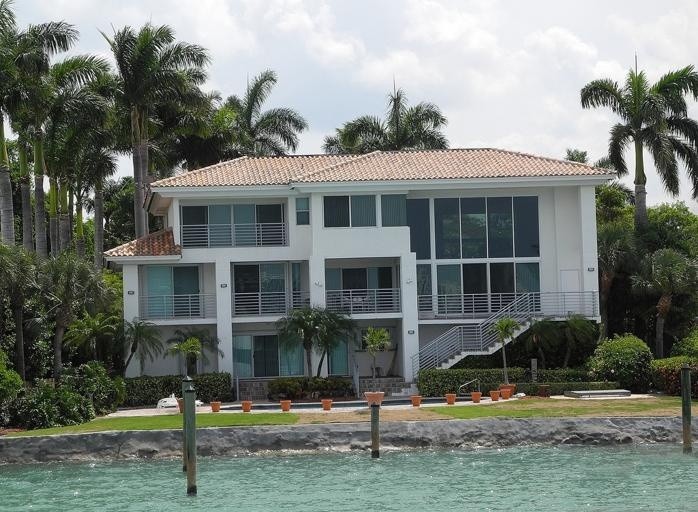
[490,389,510,401]
[470,391,482,403]
[364,391,385,409]
[411,395,422,407]
[210,401,221,412]
[321,399,332,410]
[446,393,456,404]
[280,400,291,411]
[240,400,252,412]
